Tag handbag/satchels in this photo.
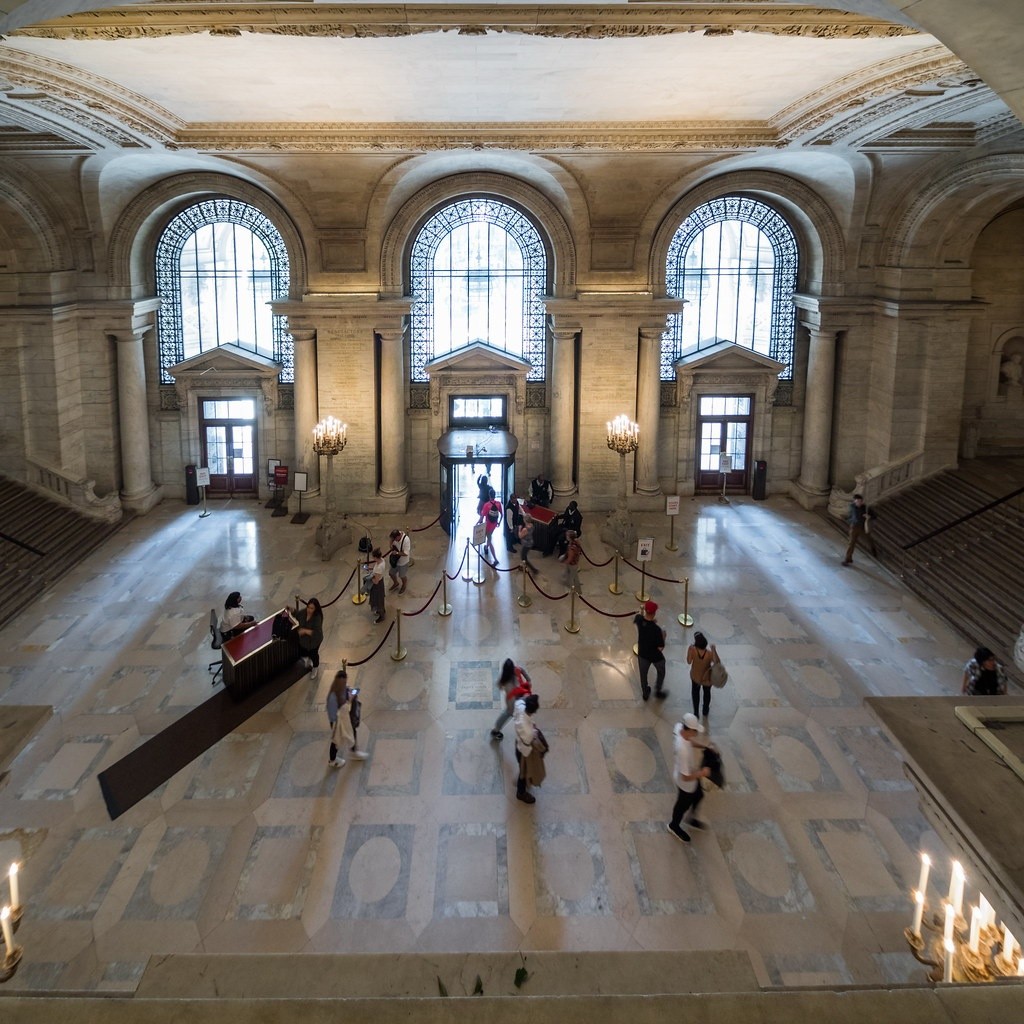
[273,607,292,637]
[711,648,728,688]
[532,730,548,753]
[473,521,486,546]
[390,552,400,568]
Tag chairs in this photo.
[208,610,230,685]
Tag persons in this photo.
[388,530,410,596]
[478,489,503,566]
[518,514,538,576]
[543,501,582,558]
[841,494,877,565]
[490,659,532,740]
[286,598,323,679]
[667,712,719,842]
[632,601,669,700]
[327,671,370,767]
[567,530,582,594]
[219,592,257,642]
[687,632,720,718]
[962,647,1008,696]
[464,464,492,475]
[504,494,525,553]
[476,474,493,523]
[514,684,547,803]
[367,548,385,623]
[528,475,554,508]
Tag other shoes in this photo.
[643,685,651,701]
[303,656,317,679]
[398,585,406,596]
[483,542,564,577]
[667,822,690,843]
[388,582,399,592]
[491,729,503,740]
[374,609,385,625]
[517,792,535,804]
[350,750,368,761]
[328,758,345,768]
[684,817,704,831]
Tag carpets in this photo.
[97,656,313,822]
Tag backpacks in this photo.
[489,500,498,522]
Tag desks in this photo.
[221,608,301,706]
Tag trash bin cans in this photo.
[185,465,199,505]
[752,460,767,500]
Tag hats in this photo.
[683,713,705,733]
[645,601,658,613]
[974,645,996,663]
